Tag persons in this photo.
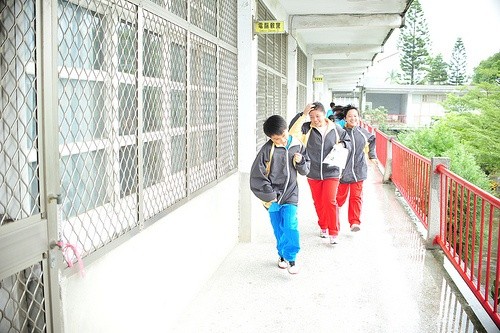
[333,107,343,114]
[335,113,344,126]
[335,104,376,232]
[327,102,335,117]
[289,102,352,244]
[250,115,311,274]
[328,115,335,122]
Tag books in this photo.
[323,146,349,169]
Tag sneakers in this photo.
[278,256,288,269]
[350,223,360,232]
[319,228,327,238]
[288,262,298,274]
[329,234,339,244]
